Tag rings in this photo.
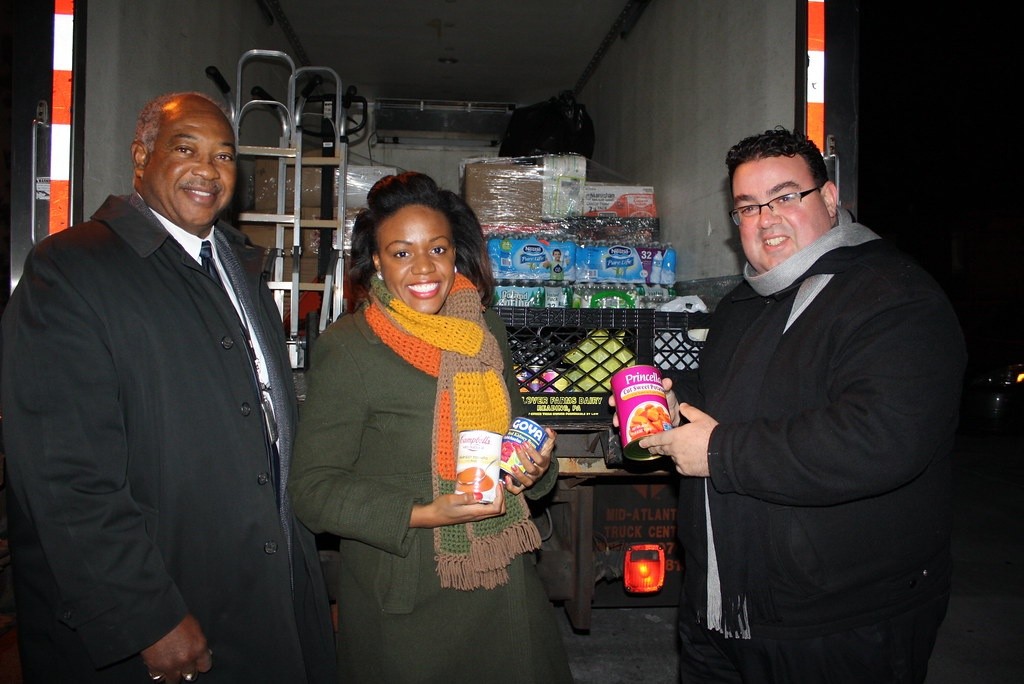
[147,671,165,681]
[182,673,199,682]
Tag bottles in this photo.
[562,251,569,267]
[650,251,662,283]
[537,291,541,305]
[492,280,675,308]
[486,231,675,279]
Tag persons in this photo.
[286,170,557,684]
[607,127,968,684]
[0,89,338,684]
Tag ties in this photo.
[200,241,281,509]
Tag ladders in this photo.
[230,43,302,371]
[285,63,347,366]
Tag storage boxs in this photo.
[479,221,562,240]
[460,164,543,226]
[238,148,397,338]
[653,312,714,379]
[562,217,660,248]
[488,306,655,424]
[583,181,654,217]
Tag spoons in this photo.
[458,458,500,484]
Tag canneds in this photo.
[498,417,549,487]
[454,429,504,504]
[610,364,672,460]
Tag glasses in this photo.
[728,187,820,225]
